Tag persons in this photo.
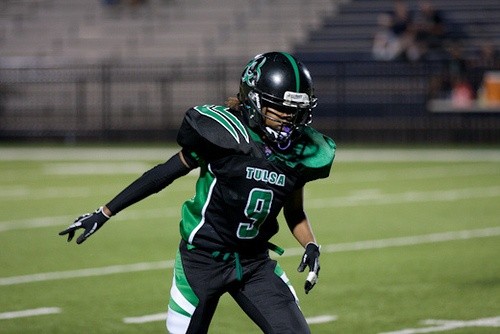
[59,52,335,334]
[371,2,444,60]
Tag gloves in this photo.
[296,242,320,294]
[58,205,110,245]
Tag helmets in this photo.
[238,51,314,143]
